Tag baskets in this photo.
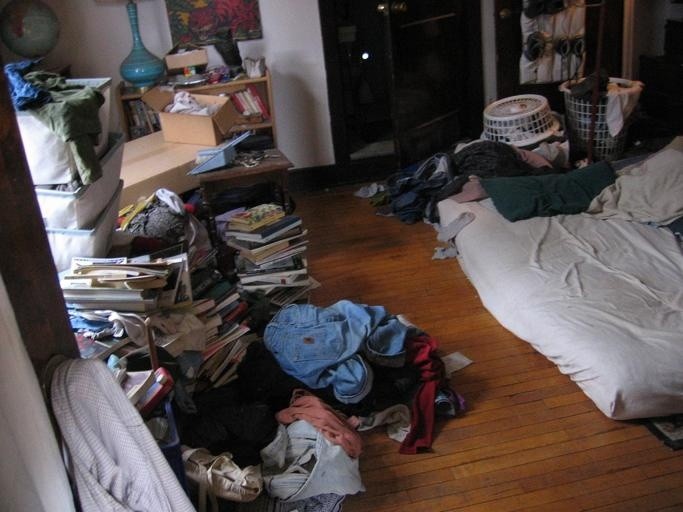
[478,94,560,149]
[561,77,642,163]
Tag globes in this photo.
[0,0,59,73]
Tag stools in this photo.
[197,147,294,282]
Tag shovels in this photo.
[186,128,253,175]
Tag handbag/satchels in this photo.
[278,391,365,504]
[179,445,262,503]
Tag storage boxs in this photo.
[120,130,214,211]
[141,85,241,146]
[14,78,125,274]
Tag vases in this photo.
[119,0,164,88]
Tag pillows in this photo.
[478,159,617,223]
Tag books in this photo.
[214,205,322,323]
[57,243,258,390]
[124,83,270,140]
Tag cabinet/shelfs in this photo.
[115,65,278,152]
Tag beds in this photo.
[437,136,683,421]
